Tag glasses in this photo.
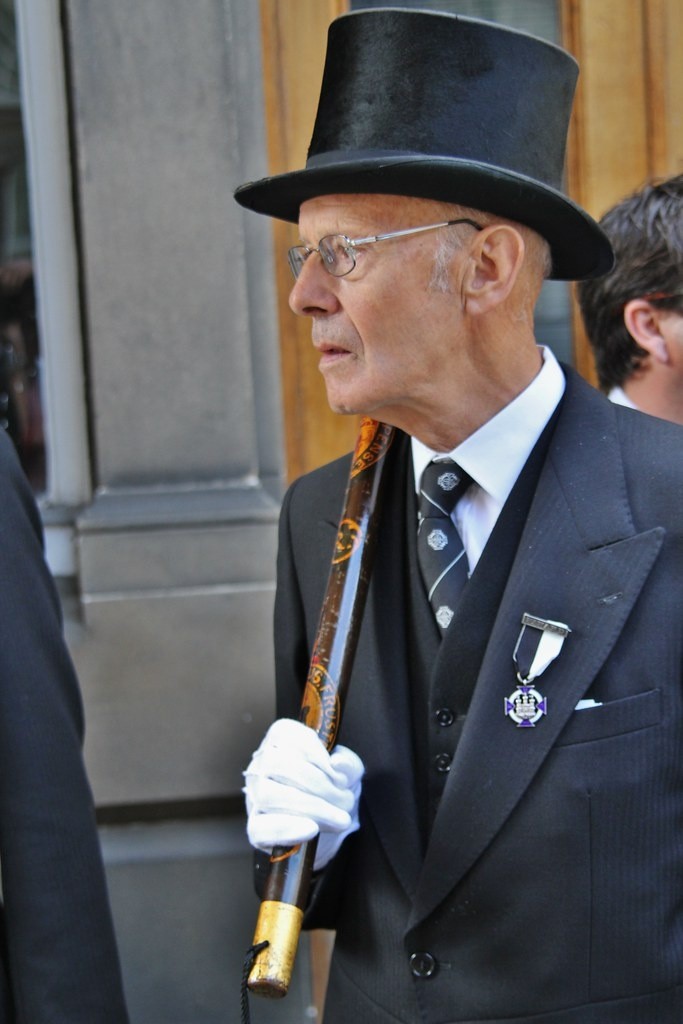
[287,218,484,282]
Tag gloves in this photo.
[239,715,364,873]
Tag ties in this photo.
[416,462,475,639]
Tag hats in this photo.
[235,6,619,282]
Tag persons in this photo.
[231,9,683,1024]
[0,427,131,1022]
[574,175,683,423]
